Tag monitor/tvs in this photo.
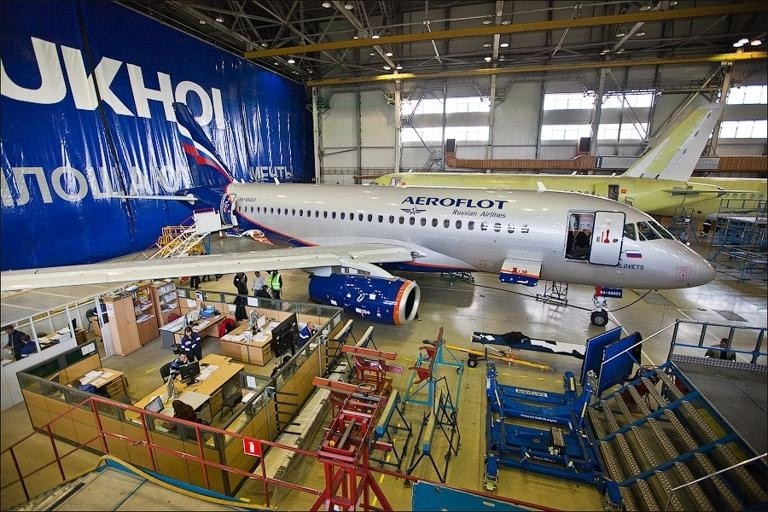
[179,361,200,385]
[271,313,300,358]
[144,396,164,415]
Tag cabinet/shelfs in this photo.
[151,281,181,328]
[125,283,159,346]
[104,295,142,357]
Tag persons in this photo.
[190,270,282,308]
[18,335,36,356]
[705,339,736,360]
[574,229,592,260]
[94,299,108,326]
[72,380,97,402]
[2,326,26,359]
[181,328,203,363]
[172,401,201,441]
[297,322,318,347]
[169,352,189,379]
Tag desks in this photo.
[52,311,327,446]
[38,326,89,351]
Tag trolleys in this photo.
[467,343,519,370]
